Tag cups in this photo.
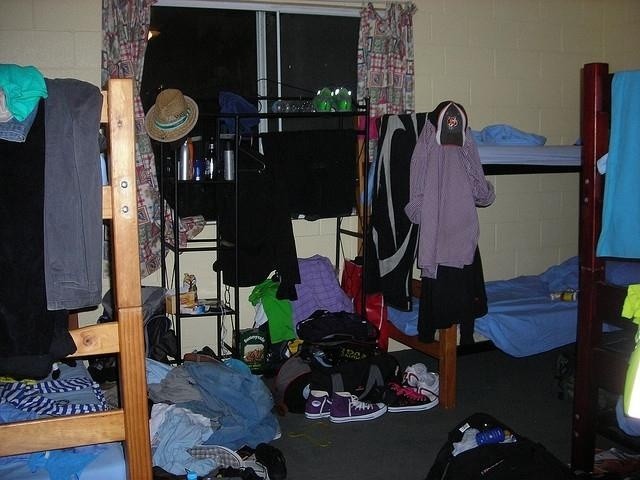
[192,304,211,314]
[179,137,235,181]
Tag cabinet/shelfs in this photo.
[157,94,373,387]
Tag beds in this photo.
[377,107,583,407]
[3,73,157,480]
[566,55,640,480]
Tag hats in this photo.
[430,99,468,149]
[142,88,200,144]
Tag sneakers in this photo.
[304,392,332,421]
[387,384,441,413]
[328,390,388,424]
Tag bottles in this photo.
[476,428,504,447]
[550,288,579,302]
[185,272,198,305]
